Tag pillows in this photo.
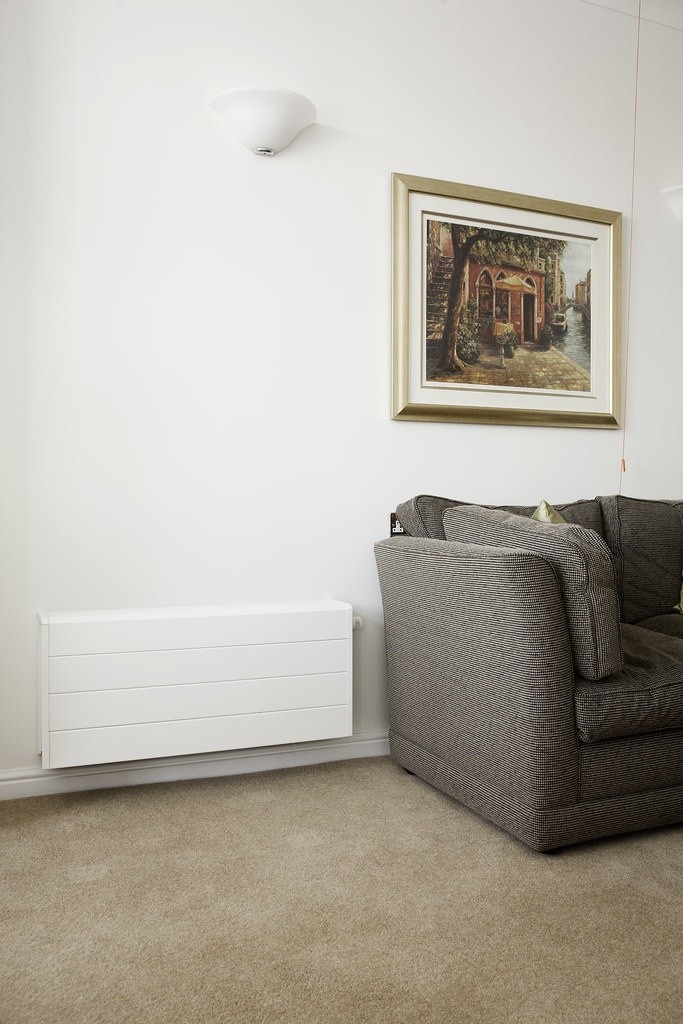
[447,502,621,683]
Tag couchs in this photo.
[374,493,683,852]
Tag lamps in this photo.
[210,86,314,155]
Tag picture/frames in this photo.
[389,172,625,430]
